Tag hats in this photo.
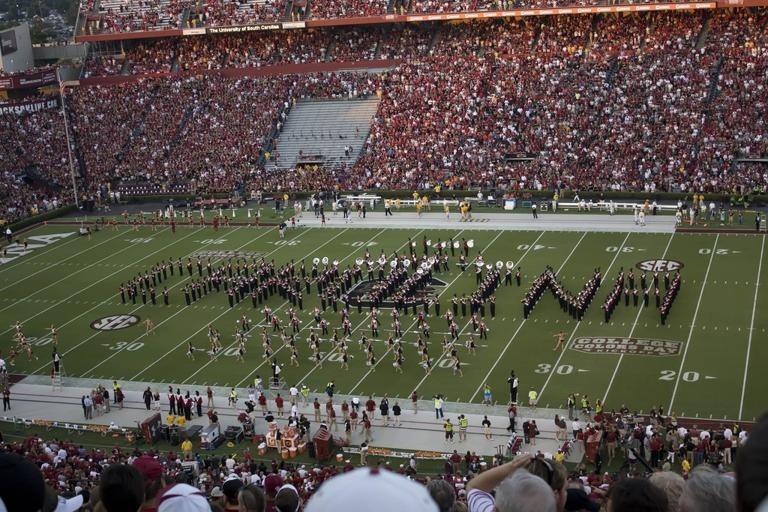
[275,483,300,512]
[302,466,439,511]
[55,494,83,511]
[211,486,224,497]
[134,456,162,478]
[0,453,47,512]
[158,484,211,512]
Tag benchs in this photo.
[261,95,382,170]
[345,195,488,207]
[557,202,682,214]
[0,416,130,438]
[243,191,275,204]
[77,0,280,32]
